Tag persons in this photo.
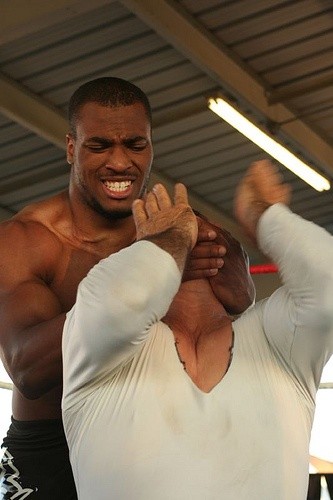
[62,159,333,500]
[1,78,226,500]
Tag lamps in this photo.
[207,95,330,193]
